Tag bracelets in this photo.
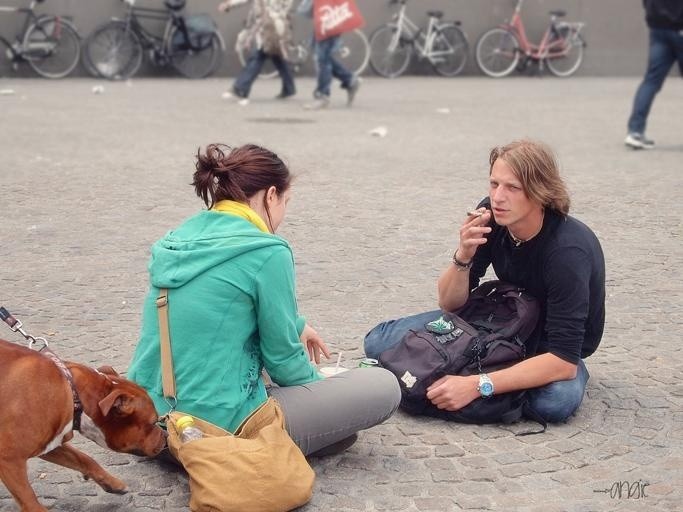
[453,249,473,270]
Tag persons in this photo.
[364,138,605,423]
[125,145,402,466]
[298,1,362,110]
[623,0,683,149]
[219,0,297,106]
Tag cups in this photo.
[319,364,347,380]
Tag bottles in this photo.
[173,416,203,445]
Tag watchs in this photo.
[477,374,494,399]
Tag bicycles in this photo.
[81,1,226,80]
[235,0,370,80]
[474,0,587,77]
[0,0,83,79]
[369,0,470,78]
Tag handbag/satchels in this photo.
[377,279,543,425]
[312,1,365,43]
[166,396,316,512]
[263,26,294,56]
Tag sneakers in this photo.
[347,78,362,106]
[623,132,655,150]
[305,98,328,110]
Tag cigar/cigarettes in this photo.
[467,211,483,216]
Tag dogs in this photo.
[0,337,170,512]
